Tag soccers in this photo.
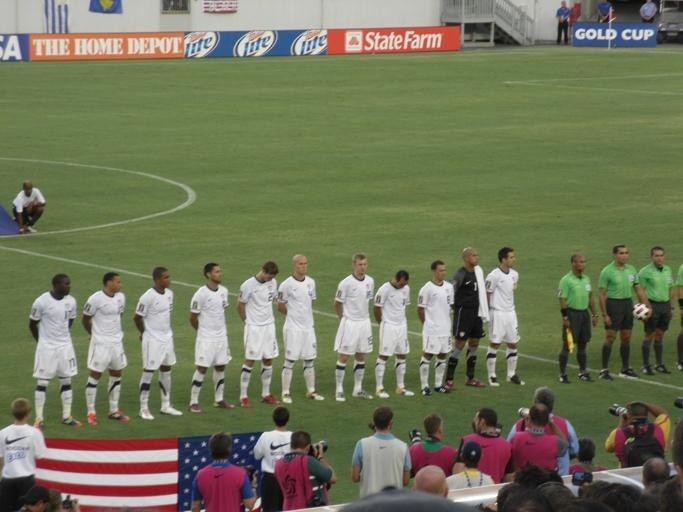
[632,304,652,321]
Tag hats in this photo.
[18,483,50,504]
[460,440,483,460]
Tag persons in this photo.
[82,272,131,427]
[277,254,323,404]
[239,261,280,408]
[597,1,614,21]
[333,253,374,402]
[486,247,526,387]
[134,268,183,420]
[417,260,454,395]
[29,274,83,430]
[639,0,658,22]
[190,263,236,413]
[0,398,81,512]
[556,244,682,385]
[374,270,413,397]
[555,1,570,45]
[14,180,46,234]
[192,388,683,512]
[444,247,486,390]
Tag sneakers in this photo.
[18,225,37,234]
[87,412,98,425]
[559,374,568,383]
[641,365,654,375]
[34,417,43,428]
[376,386,389,398]
[434,384,449,393]
[335,388,346,402]
[160,404,182,415]
[466,378,486,387]
[654,364,670,373]
[421,387,431,395]
[282,394,292,403]
[215,400,235,409]
[599,369,614,381]
[189,403,201,412]
[260,394,279,404]
[396,387,415,396]
[139,408,154,419]
[576,372,591,381]
[351,390,372,399]
[675,362,682,370]
[506,374,525,386]
[107,409,130,422]
[489,376,500,387]
[305,391,324,401]
[618,367,639,378]
[445,380,453,390]
[239,397,249,410]
[61,416,82,426]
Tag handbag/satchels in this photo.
[304,490,326,507]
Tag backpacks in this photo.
[625,435,665,467]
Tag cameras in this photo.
[608,403,627,419]
[674,398,683,409]
[572,471,593,485]
[518,407,530,418]
[63,495,76,508]
[408,429,422,444]
[245,464,255,480]
[308,439,328,456]
